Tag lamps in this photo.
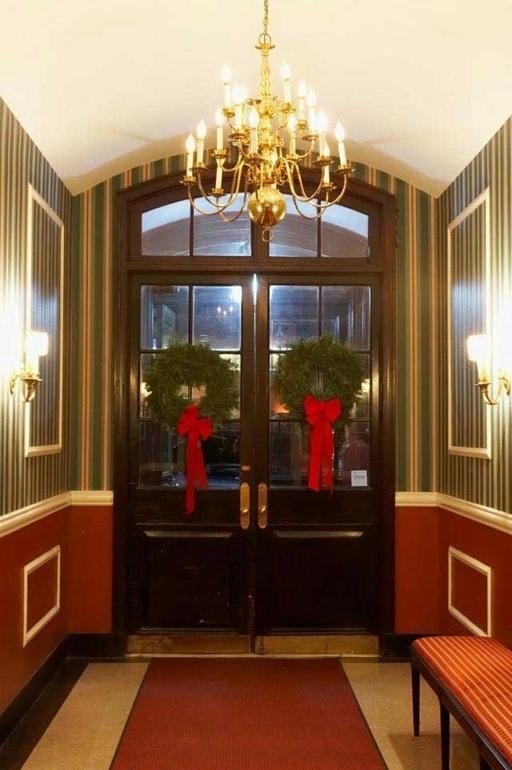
[463,328,512,412]
[179,0,355,243]
[4,326,55,408]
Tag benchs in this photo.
[407,636,511,769]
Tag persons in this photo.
[341,416,369,481]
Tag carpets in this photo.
[108,656,389,770]
